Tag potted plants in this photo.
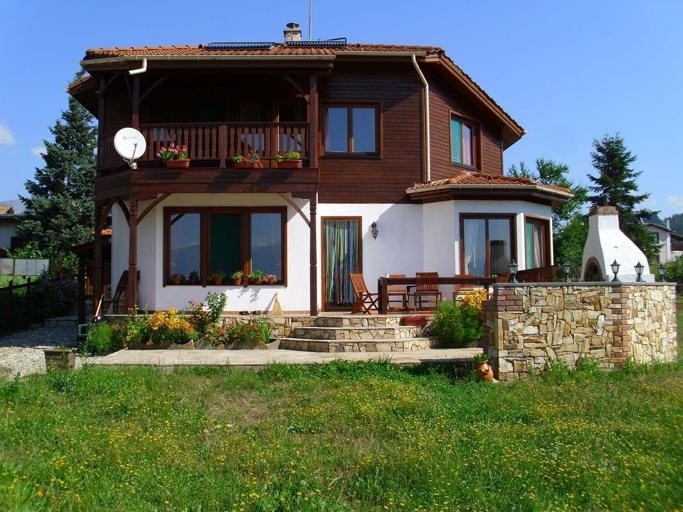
[189,270,265,285]
[274,150,301,168]
[474,353,494,381]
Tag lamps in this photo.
[561,260,573,283]
[507,260,520,283]
[608,258,666,283]
[371,221,379,241]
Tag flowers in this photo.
[231,153,259,165]
[171,272,183,280]
[155,142,188,160]
[264,274,276,282]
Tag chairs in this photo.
[348,272,511,315]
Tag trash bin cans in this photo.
[43,348,77,374]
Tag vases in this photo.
[268,281,272,285]
[165,159,190,168]
[235,160,258,168]
[173,280,180,285]
